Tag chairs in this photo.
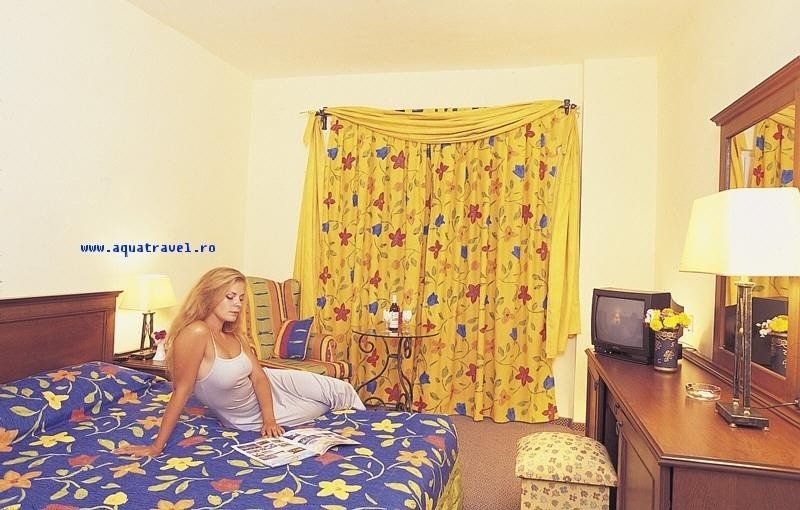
[235,277,352,384]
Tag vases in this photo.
[770,333,787,377]
[654,330,679,374]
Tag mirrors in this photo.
[710,56,800,407]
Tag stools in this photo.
[516,432,619,510]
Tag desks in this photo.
[351,328,440,414]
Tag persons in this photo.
[106,265,367,464]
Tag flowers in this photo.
[641,309,691,333]
[756,313,789,339]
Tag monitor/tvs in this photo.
[591,286,684,366]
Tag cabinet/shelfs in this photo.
[584,348,800,510]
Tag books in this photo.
[230,423,362,469]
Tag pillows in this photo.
[273,316,313,361]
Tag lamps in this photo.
[679,187,800,429]
[118,274,178,360]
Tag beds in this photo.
[0,290,456,510]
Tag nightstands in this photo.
[115,355,173,382]
